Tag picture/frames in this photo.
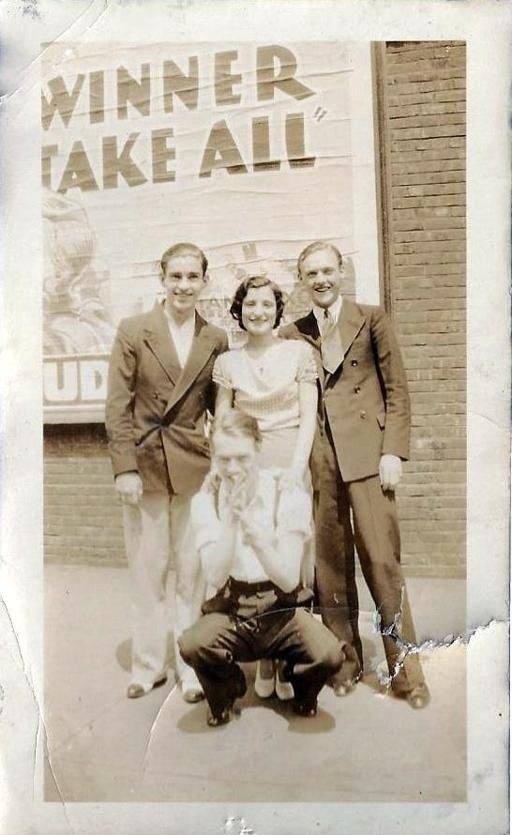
[39,42,392,427]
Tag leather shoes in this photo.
[335,676,356,697]
[127,676,167,698]
[292,698,317,718]
[182,689,204,702]
[254,657,275,698]
[407,681,431,710]
[276,668,295,701]
[207,672,248,726]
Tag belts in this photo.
[230,576,273,593]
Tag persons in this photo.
[175,403,345,728]
[208,272,321,701]
[105,241,234,708]
[275,240,432,713]
